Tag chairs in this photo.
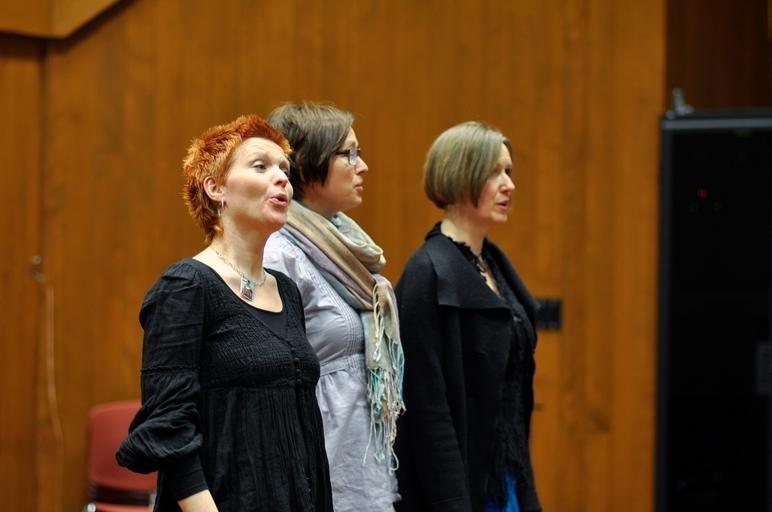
[84,402,157,512]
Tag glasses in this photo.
[335,146,362,166]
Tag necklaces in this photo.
[210,246,266,302]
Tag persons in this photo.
[263,100,407,512]
[114,115,333,512]
[392,120,541,512]
